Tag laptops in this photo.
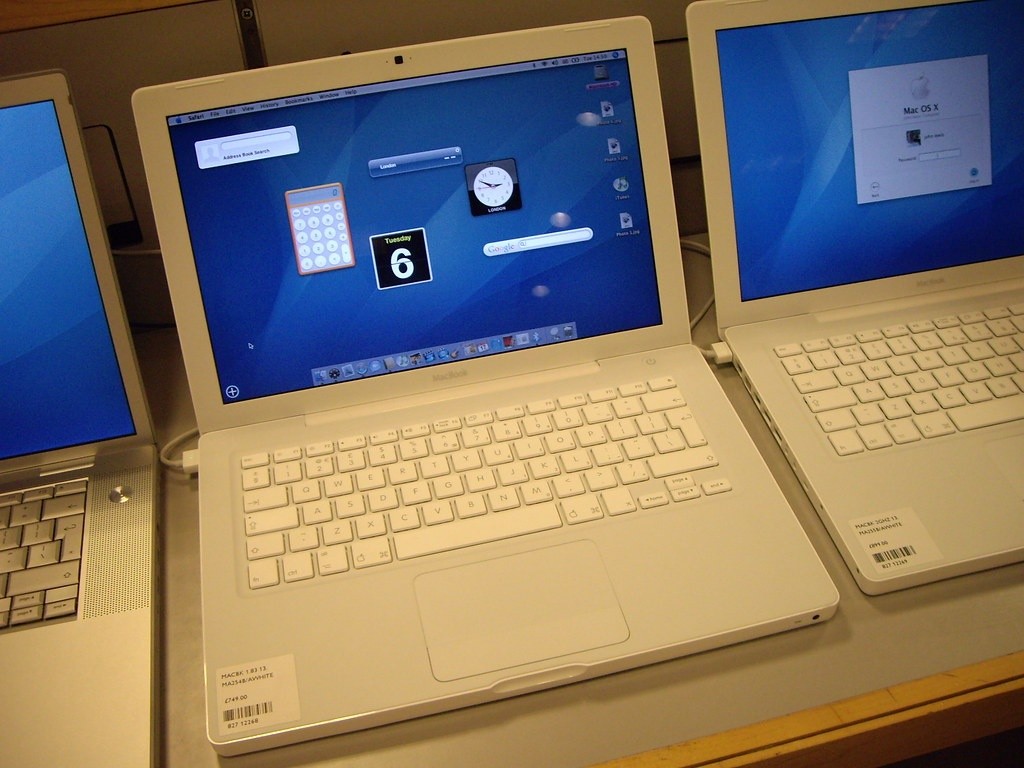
[684,0,1024,598]
[128,16,845,759]
[1,66,162,768]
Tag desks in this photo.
[96,229,1024,768]
